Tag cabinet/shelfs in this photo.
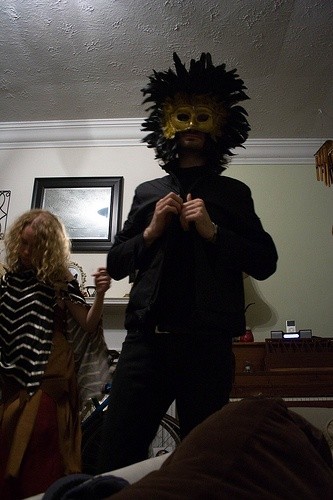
[232,337,333,408]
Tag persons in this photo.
[0,210,111,500]
[98,94,278,474]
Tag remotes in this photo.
[286,320,295,333]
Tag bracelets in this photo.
[211,221,218,244]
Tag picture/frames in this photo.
[30,176,124,252]
[66,261,87,294]
